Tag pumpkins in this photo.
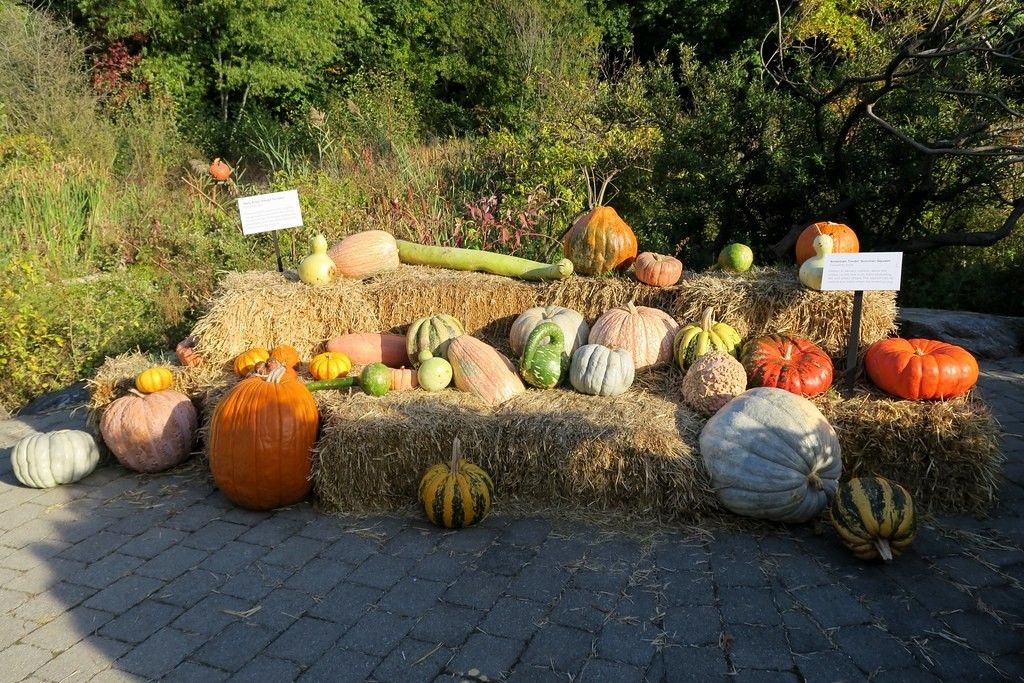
[209,161,230,180]
[10,230,574,529]
[508,206,978,562]
